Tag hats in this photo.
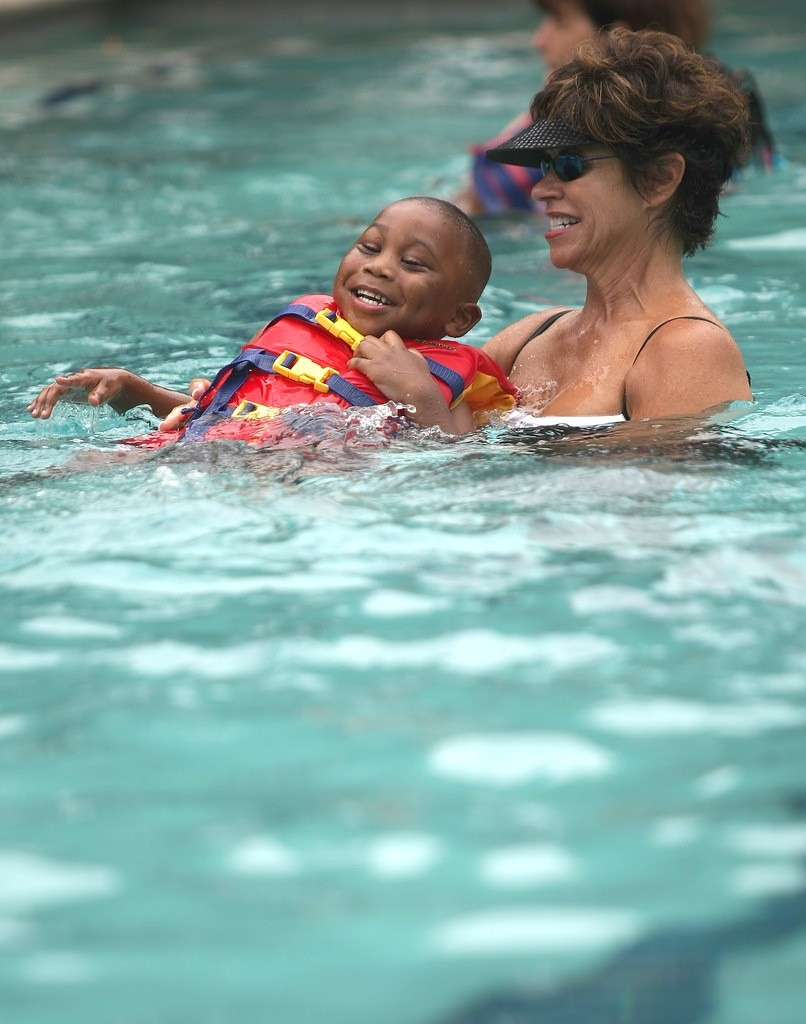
[486,113,600,168]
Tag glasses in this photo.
[536,148,619,183]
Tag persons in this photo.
[157,27,757,434]
[25,196,523,435]
[443,0,778,216]
